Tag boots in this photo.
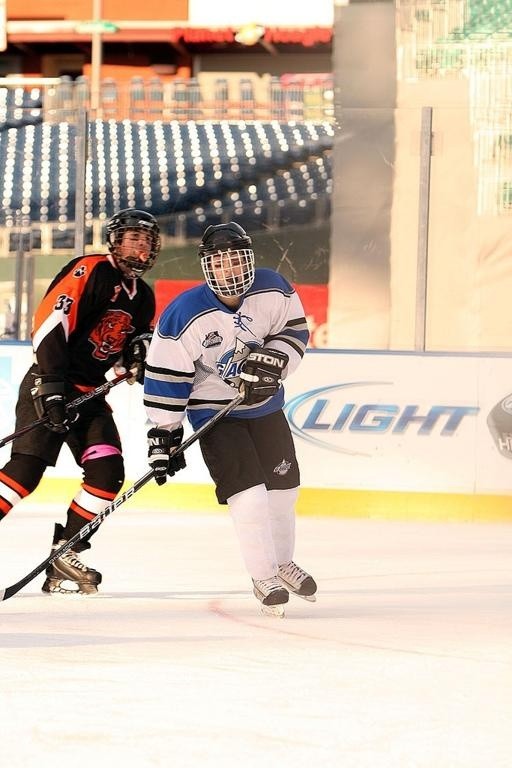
[46,523,102,584]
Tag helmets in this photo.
[197,221,255,299]
[105,207,161,280]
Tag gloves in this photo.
[30,374,80,434]
[238,347,289,406]
[147,425,186,486]
[122,338,153,385]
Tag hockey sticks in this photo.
[0,391,247,600]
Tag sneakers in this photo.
[253,575,289,605]
[273,560,317,597]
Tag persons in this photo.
[141,221,318,607]
[1,208,165,588]
